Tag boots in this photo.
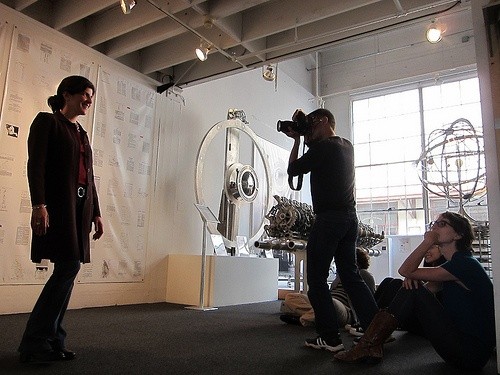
[334,311,398,362]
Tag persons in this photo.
[15,75,103,360]
[345,243,446,337]
[333,210,495,372]
[280,246,376,326]
[287,107,394,351]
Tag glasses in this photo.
[429,220,459,235]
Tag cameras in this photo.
[277,108,313,136]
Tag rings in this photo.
[37,222,40,225]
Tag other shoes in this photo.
[280,313,300,324]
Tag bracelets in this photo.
[32,205,47,209]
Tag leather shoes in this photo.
[52,344,77,359]
[20,348,66,363]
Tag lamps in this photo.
[196,41,214,61]
[425,21,447,45]
[262,65,276,81]
[120,0,137,15]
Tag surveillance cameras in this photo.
[157,82,174,94]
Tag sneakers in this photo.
[353,332,394,345]
[344,320,360,330]
[349,327,365,335]
[305,332,344,350]
